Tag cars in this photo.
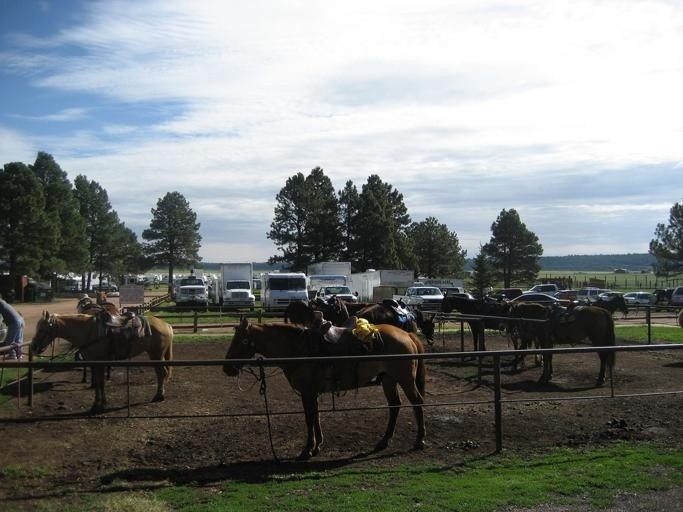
[92,282,119,292]
[441,283,683,313]
[24,283,54,302]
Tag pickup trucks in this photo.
[392,286,445,310]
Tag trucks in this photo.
[173,261,358,312]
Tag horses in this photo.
[441,294,629,387]
[28,288,174,415]
[223,295,436,458]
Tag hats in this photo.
[80,294,90,300]
